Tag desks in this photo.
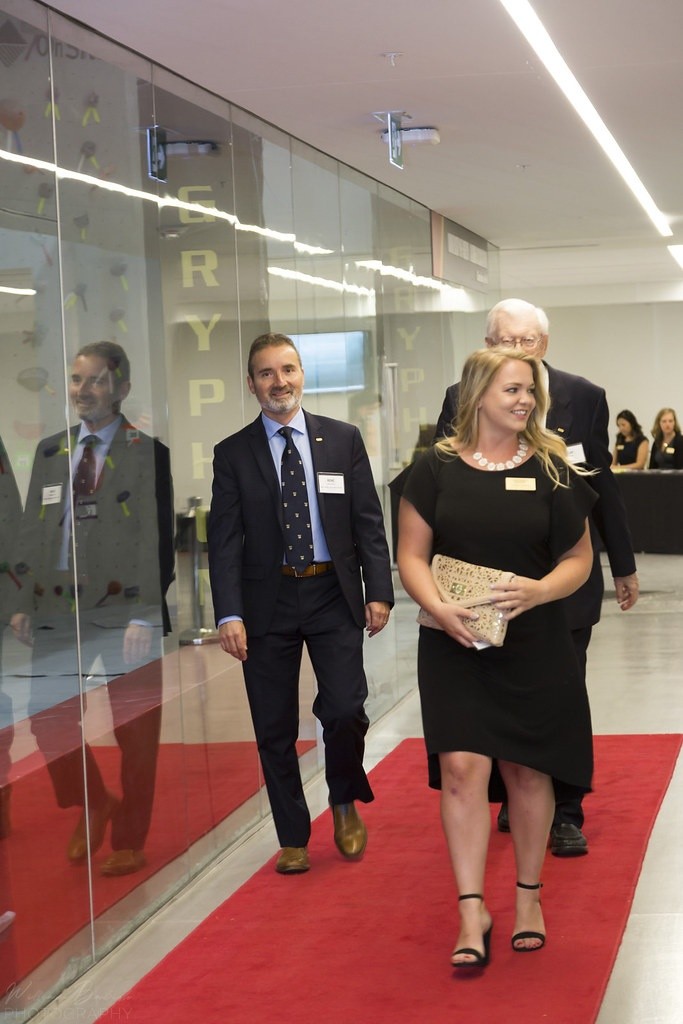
[591,470,683,554]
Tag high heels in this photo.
[511,882,545,951]
[450,894,493,966]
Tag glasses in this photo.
[494,335,541,350]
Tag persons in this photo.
[10,342,178,877]
[432,298,640,856]
[206,333,397,872]
[610,410,649,472]
[649,409,683,470]
[388,347,595,969]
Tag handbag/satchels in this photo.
[414,554,517,649]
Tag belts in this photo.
[281,561,335,578]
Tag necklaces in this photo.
[472,434,529,470]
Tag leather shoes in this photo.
[100,848,145,874]
[332,800,366,859]
[275,845,311,872]
[69,791,118,862]
[496,802,508,833]
[550,816,589,856]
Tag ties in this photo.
[276,427,315,575]
[67,435,100,600]
